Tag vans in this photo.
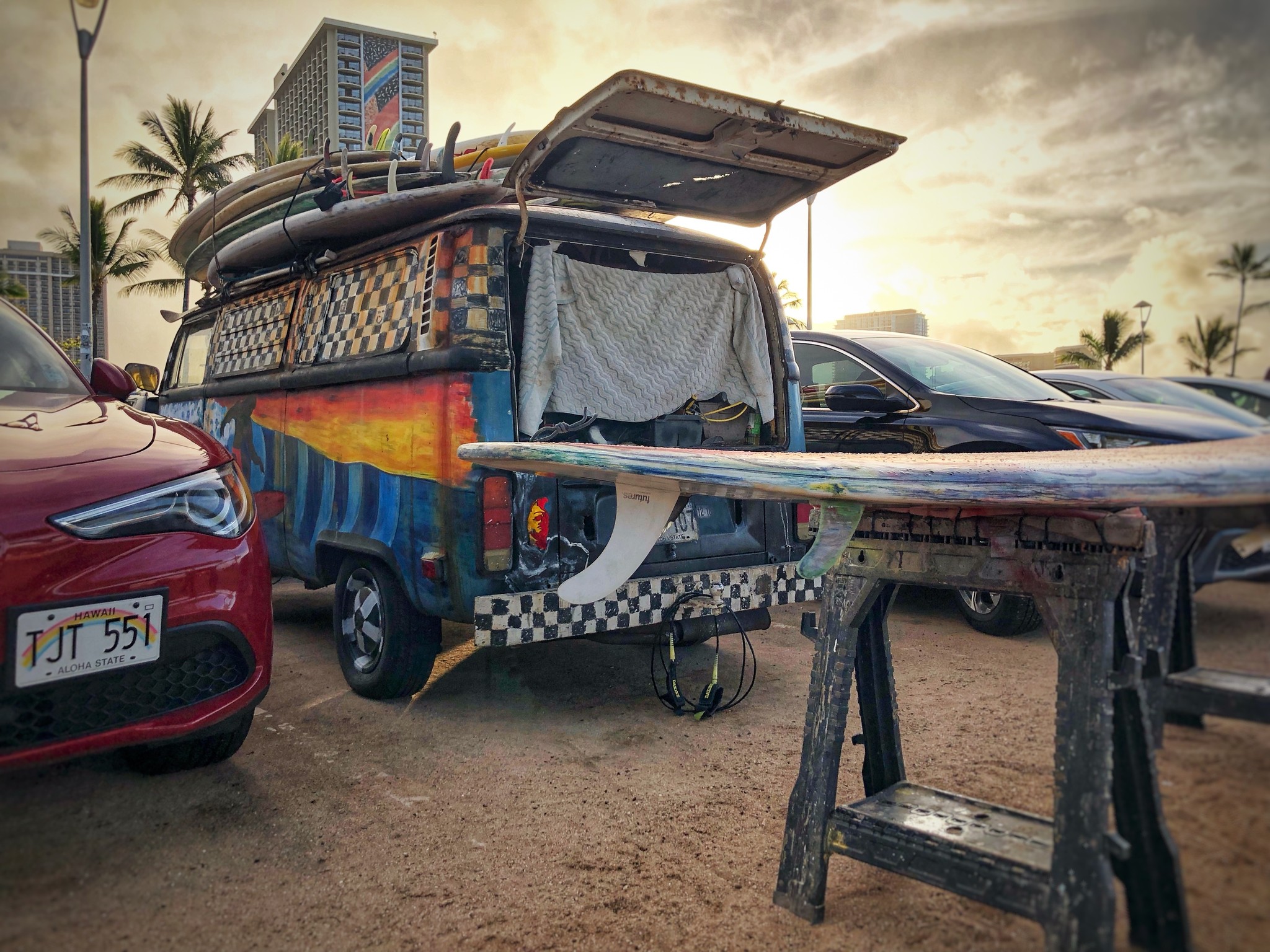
[136,69,906,700]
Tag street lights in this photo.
[804,192,817,332]
[1133,299,1152,376]
[69,0,108,385]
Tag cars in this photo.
[792,329,1269,639]
[1,296,275,776]
